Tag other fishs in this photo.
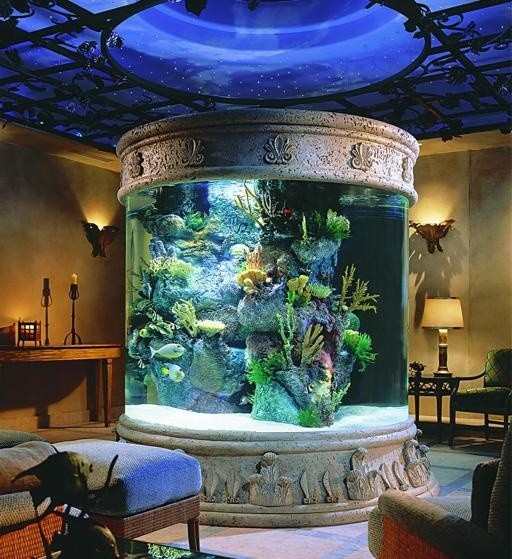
[8,451,94,492]
[149,342,187,358]
[49,523,120,559]
[161,362,185,383]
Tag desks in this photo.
[0,342,122,427]
[409,373,456,423]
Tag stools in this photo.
[52,439,203,553]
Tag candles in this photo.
[70,272,77,284]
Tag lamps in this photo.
[80,220,118,257]
[408,219,456,254]
[419,295,464,376]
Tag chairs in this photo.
[449,347,511,446]
[0,430,66,558]
[367,426,511,557]
